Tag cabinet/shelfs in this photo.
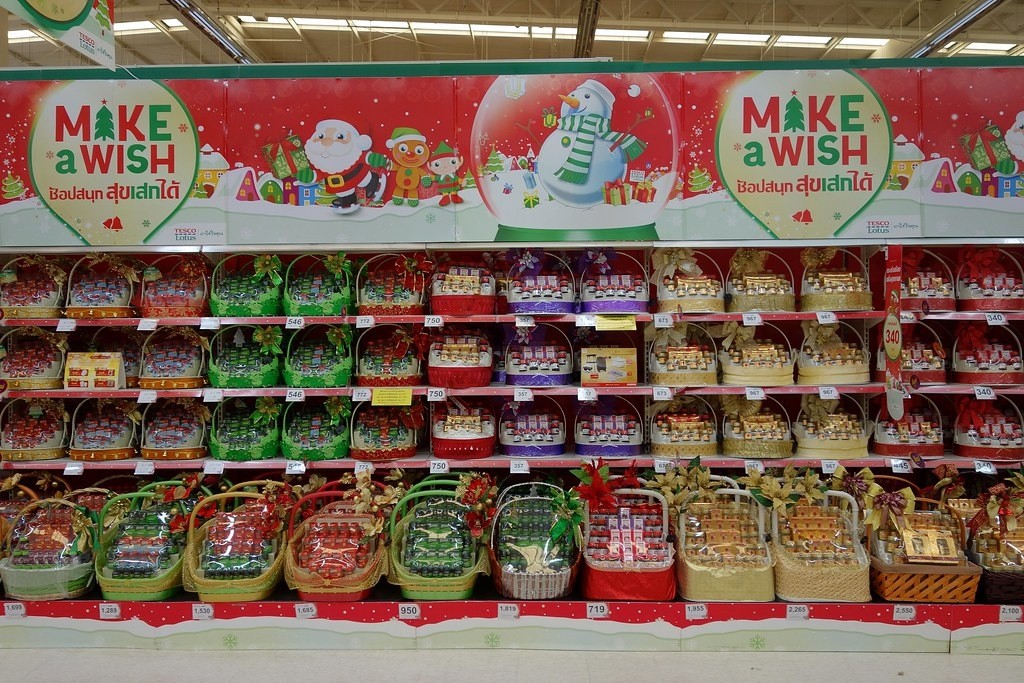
[0,238,1024,655]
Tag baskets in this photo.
[0,246,1024,602]
